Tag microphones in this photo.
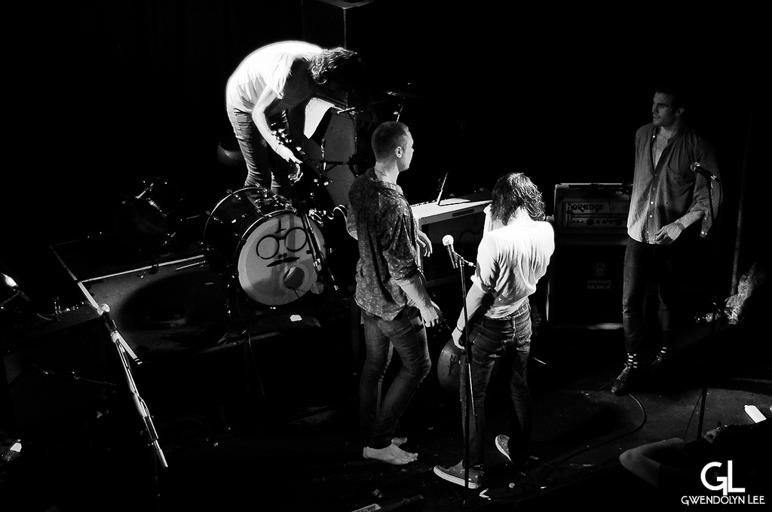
[690,161,721,183]
[442,235,459,269]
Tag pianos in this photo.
[410,189,494,226]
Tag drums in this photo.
[204,187,325,307]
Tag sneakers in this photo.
[431,458,483,489]
[494,432,521,468]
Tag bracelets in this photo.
[456,326,463,333]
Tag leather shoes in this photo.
[609,361,645,397]
[647,349,672,374]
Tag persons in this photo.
[348,120,444,466]
[432,172,556,490]
[610,90,726,395]
[224,39,365,198]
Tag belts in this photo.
[499,303,529,320]
